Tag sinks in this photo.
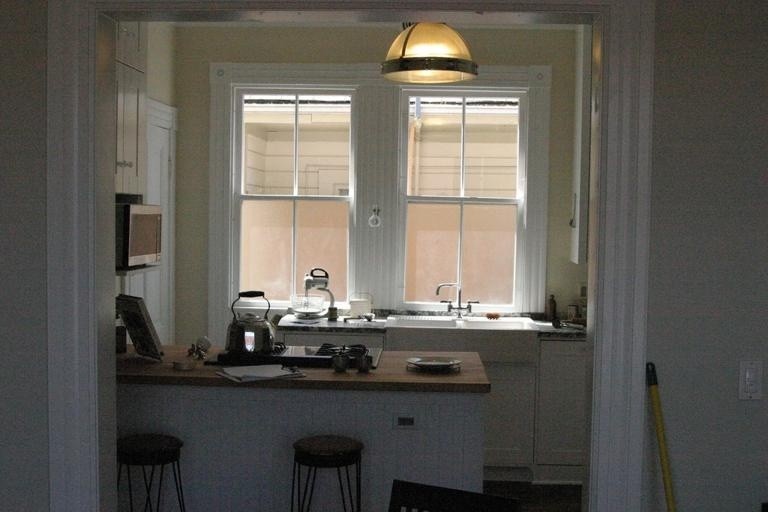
[384,314,464,329]
[455,316,540,331]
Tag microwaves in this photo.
[115,202,163,268]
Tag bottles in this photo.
[545,295,555,321]
[328,307,336,321]
[116,326,126,353]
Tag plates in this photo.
[405,357,462,372]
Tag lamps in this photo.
[367,205,383,227]
[377,21,479,83]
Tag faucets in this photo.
[435,283,462,319]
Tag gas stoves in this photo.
[203,345,382,370]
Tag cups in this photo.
[363,313,375,321]
[351,355,372,373]
[567,305,586,322]
[333,354,349,373]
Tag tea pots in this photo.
[225,290,285,355]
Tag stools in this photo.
[114,432,186,510]
[291,433,365,511]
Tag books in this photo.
[116,293,163,360]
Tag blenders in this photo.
[288,268,334,324]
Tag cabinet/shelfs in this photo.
[114,21,149,196]
[480,365,536,468]
[536,332,590,470]
[567,25,590,265]
